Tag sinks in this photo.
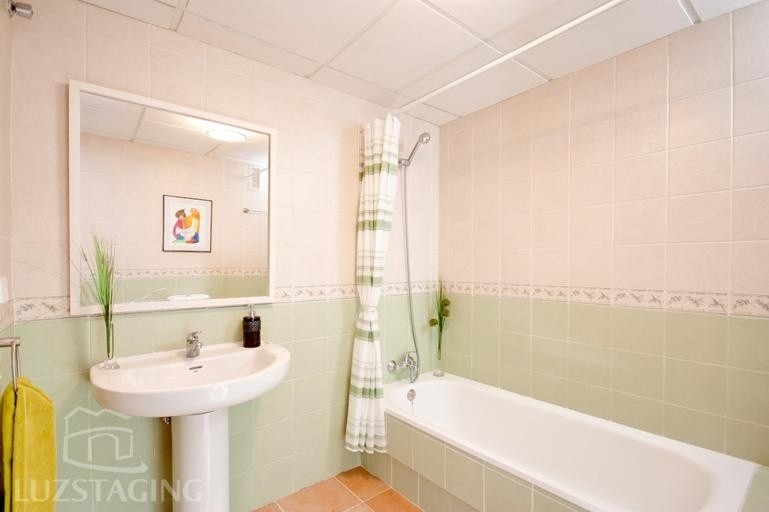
[89,340,290,417]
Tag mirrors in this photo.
[66,73,277,318]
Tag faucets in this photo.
[396,351,419,371]
[186,332,202,358]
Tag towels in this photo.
[0,375,58,511]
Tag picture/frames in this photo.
[162,193,213,254]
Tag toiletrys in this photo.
[243,303,260,348]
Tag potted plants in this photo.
[69,228,120,371]
[423,281,450,375]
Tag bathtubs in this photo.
[379,371,760,512]
